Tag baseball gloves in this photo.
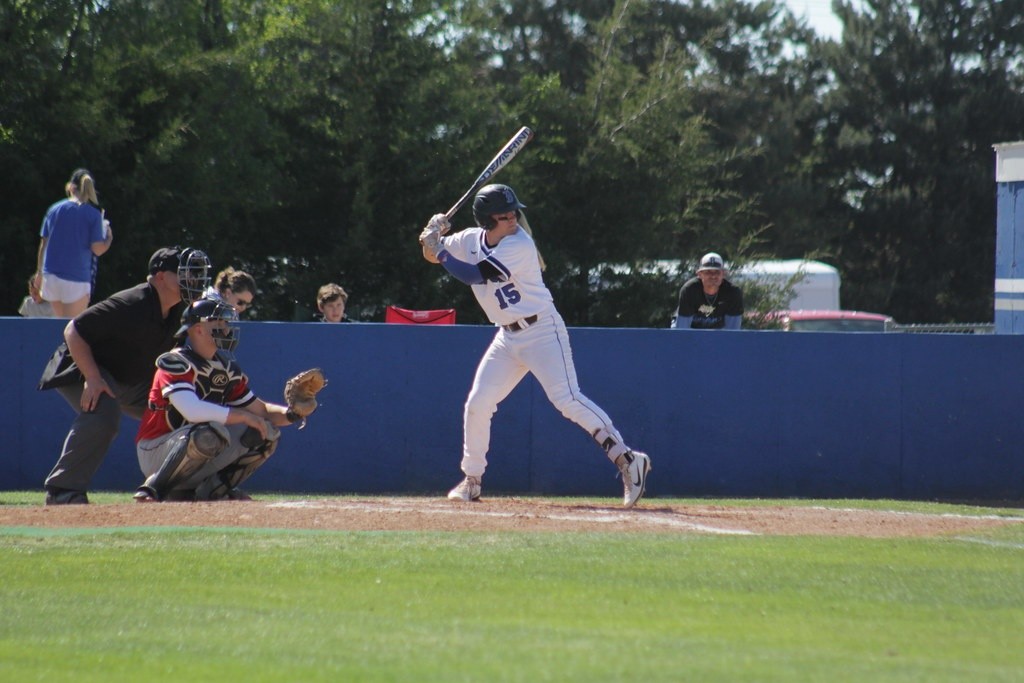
[284,366,329,433]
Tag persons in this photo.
[420,184,651,508]
[132,299,329,502]
[315,284,354,322]
[668,252,744,331]
[37,246,211,505]
[19,168,112,319]
[201,266,259,320]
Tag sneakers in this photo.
[617,451,652,508]
[448,475,480,501]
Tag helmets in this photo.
[175,299,230,336]
[473,184,526,230]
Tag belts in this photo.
[501,315,537,332]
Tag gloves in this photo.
[420,225,441,253]
[428,213,451,236]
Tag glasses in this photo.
[230,289,251,308]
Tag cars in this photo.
[749,310,890,332]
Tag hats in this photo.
[149,247,203,272]
[699,252,723,270]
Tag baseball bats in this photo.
[419,124,534,246]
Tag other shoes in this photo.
[46,488,88,505]
